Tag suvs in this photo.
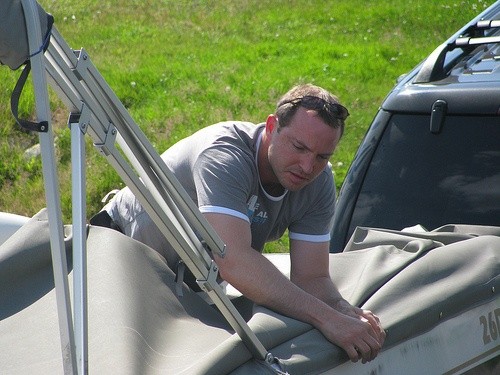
[330,1,500,255]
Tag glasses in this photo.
[277,95,349,120]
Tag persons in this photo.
[88,82,387,365]
